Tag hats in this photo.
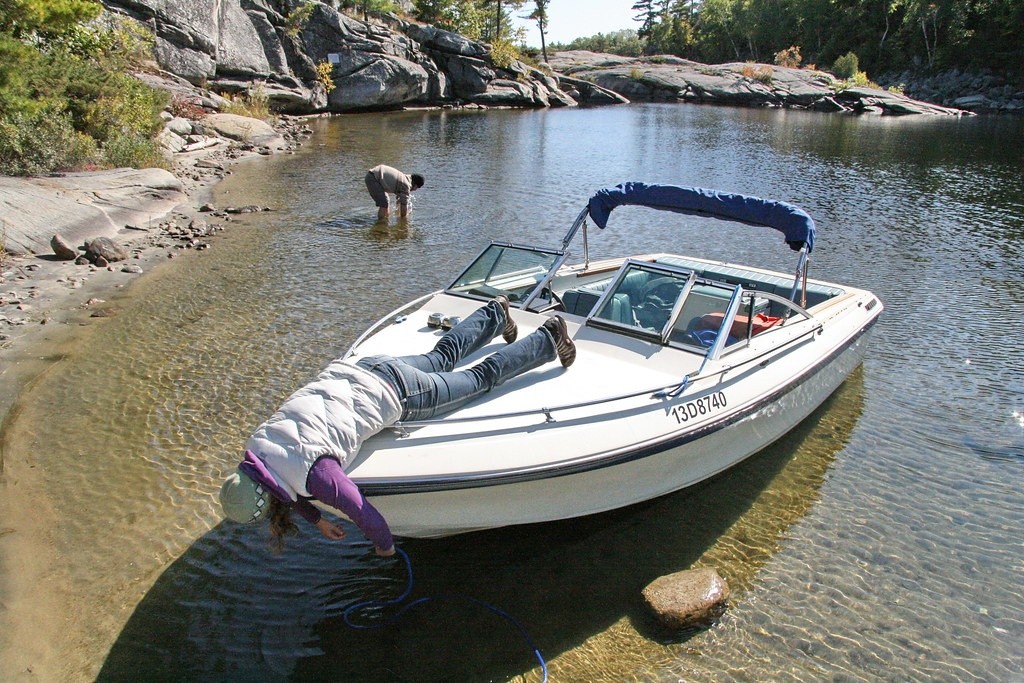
[412,174,424,188]
[220,467,273,525]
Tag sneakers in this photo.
[543,315,577,368]
[492,294,518,344]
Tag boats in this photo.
[296,181,886,544]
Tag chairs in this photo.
[563,291,622,322]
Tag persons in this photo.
[219,295,576,557]
[366,165,425,218]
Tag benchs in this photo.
[570,270,661,327]
